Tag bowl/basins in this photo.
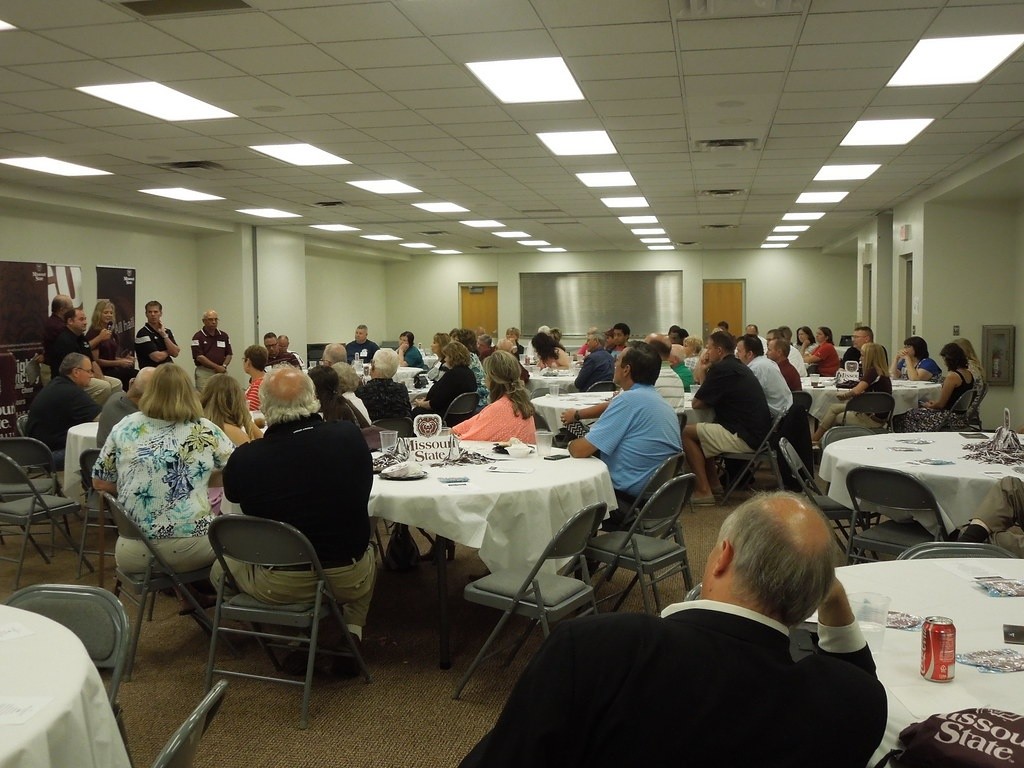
[505,446,531,458]
[381,463,409,476]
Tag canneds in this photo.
[920,616,956,682]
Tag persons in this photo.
[23,293,156,471]
[198,373,264,515]
[191,310,233,390]
[210,364,376,676]
[134,300,180,368]
[91,363,237,596]
[957,475,1024,558]
[457,490,889,768]
[243,321,985,560]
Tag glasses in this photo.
[72,366,92,375]
[851,334,869,341]
[265,341,278,348]
[242,356,249,361]
[319,357,333,365]
[204,318,218,322]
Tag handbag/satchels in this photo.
[385,522,418,570]
[563,420,590,438]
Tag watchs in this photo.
[574,411,580,420]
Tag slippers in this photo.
[684,497,717,507]
[710,486,723,497]
[179,597,225,615]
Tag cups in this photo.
[548,383,559,396]
[690,385,700,396]
[534,431,554,456]
[846,591,890,655]
[309,361,317,368]
[253,411,265,427]
[809,374,820,386]
[379,430,399,452]
[438,427,451,435]
[519,354,527,364]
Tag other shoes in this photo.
[334,632,360,675]
[283,656,316,675]
[811,441,820,449]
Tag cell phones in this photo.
[544,455,570,460]
[107,321,113,331]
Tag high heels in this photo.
[420,537,455,561]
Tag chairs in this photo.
[0,379,1021,768]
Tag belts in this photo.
[261,552,364,571]
[866,412,887,424]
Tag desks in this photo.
[800,377,944,433]
[62,421,105,510]
[0,603,132,768]
[531,391,694,436]
[363,440,618,671]
[819,432,1024,566]
[806,557,1023,768]
